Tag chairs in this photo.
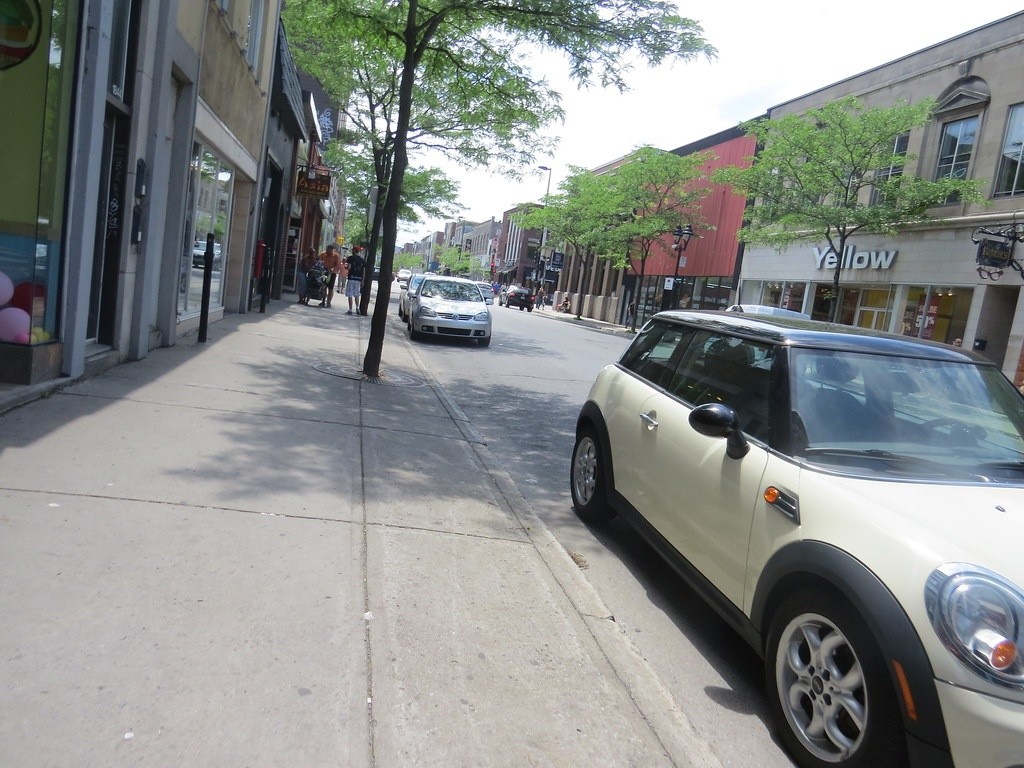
[704,341,767,421]
[811,356,864,425]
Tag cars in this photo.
[398,274,432,322]
[406,275,492,347]
[498,285,534,312]
[193,241,222,270]
[471,281,494,301]
[397,269,411,282]
[372,267,395,283]
[570,308,1024,768]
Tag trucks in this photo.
[412,266,422,276]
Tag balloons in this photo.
[11,283,47,315]
[0,272,14,306]
[16,327,50,345]
[0,307,30,344]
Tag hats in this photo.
[352,245,363,251]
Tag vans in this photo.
[702,304,810,375]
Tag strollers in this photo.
[301,265,330,308]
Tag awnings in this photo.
[496,265,517,274]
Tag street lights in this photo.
[536,165,552,285]
[670,225,694,309]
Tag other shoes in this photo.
[337,290,344,294]
[345,309,361,315]
[319,302,331,307]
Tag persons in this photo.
[486,281,514,299]
[344,247,366,314]
[296,247,317,305]
[336,257,348,294]
[557,297,571,312]
[536,287,554,309]
[625,298,639,328]
[952,338,962,347]
[320,245,340,306]
